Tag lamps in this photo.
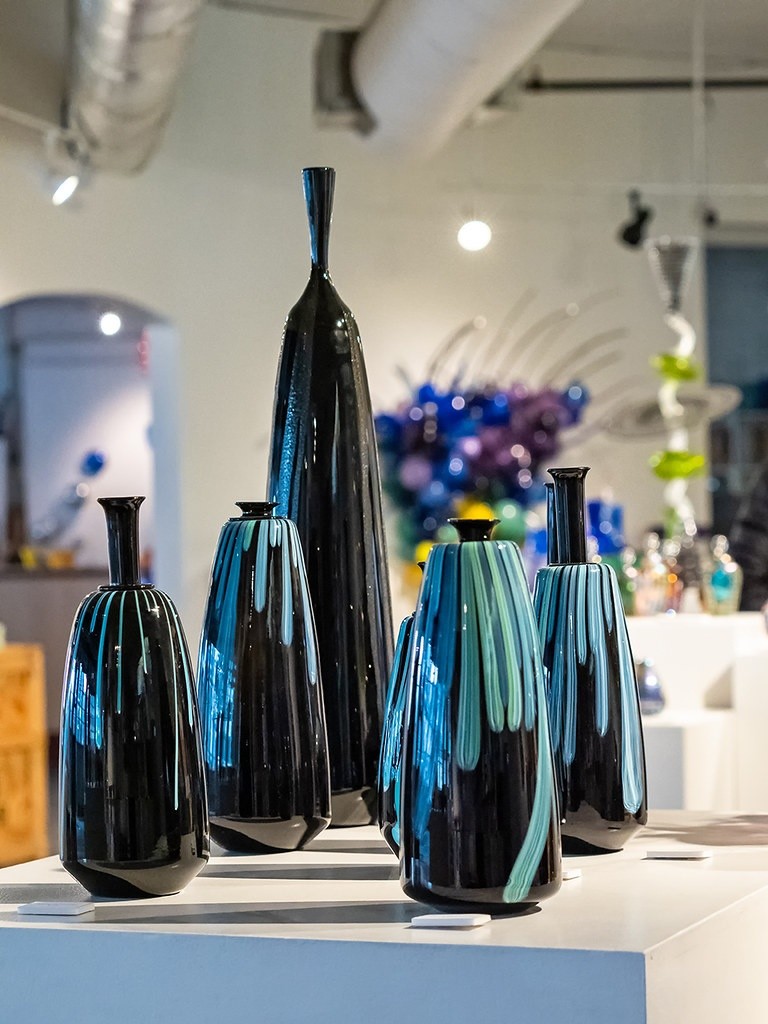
[34,131,80,208]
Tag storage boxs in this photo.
[0,643,48,869]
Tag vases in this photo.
[199,502,334,857]
[58,496,212,904]
[532,465,651,856]
[400,519,562,918]
[374,561,427,858]
[265,164,395,831]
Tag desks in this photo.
[0,809,768,1024]
[622,613,768,709]
[642,708,768,813]
